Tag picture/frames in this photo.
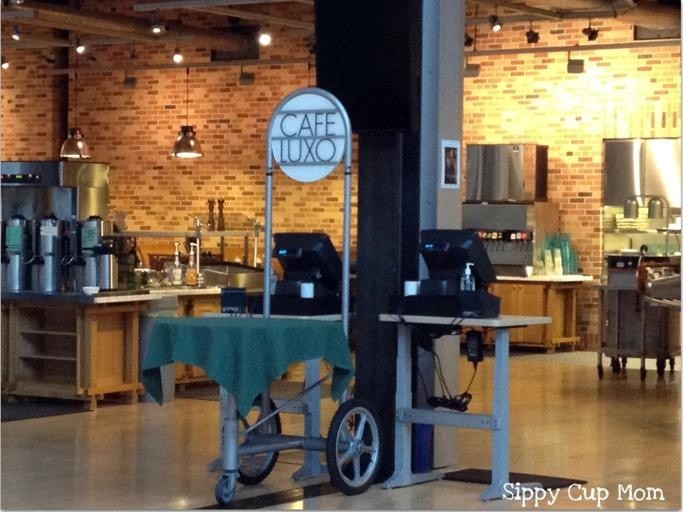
[441,139,460,188]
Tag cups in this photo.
[544,233,577,273]
[526,266,533,277]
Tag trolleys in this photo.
[598,195,679,381]
[148,87,383,506]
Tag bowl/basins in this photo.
[82,287,99,295]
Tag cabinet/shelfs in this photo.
[460,275,595,353]
[148,288,223,393]
[9,292,164,411]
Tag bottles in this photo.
[173,255,182,286]
[185,254,197,286]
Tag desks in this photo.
[379,314,553,502]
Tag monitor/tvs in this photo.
[416,227,496,283]
[268,233,348,282]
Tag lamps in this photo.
[464,56,480,77]
[59,41,92,158]
[13,28,20,42]
[582,15,599,41]
[75,38,86,53]
[168,67,204,157]
[464,34,474,47]
[567,48,584,73]
[145,8,169,36]
[2,59,9,70]
[490,4,502,33]
[525,19,539,43]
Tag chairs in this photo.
[594,285,681,382]
